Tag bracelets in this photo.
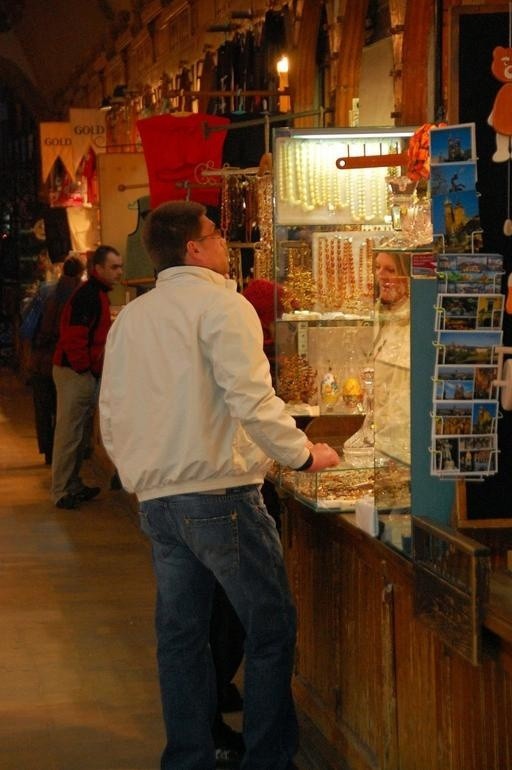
[295,454,316,475]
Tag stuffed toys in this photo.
[487,46,512,162]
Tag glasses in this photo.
[198,227,224,243]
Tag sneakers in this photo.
[211,729,247,763]
[53,496,82,511]
[223,697,248,715]
[80,487,100,500]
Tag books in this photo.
[434,254,503,473]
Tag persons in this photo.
[208,279,314,768]
[30,256,95,470]
[99,201,341,770]
[343,249,412,468]
[48,244,124,513]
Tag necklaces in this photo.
[316,235,406,302]
[220,175,314,313]
[279,136,398,223]
